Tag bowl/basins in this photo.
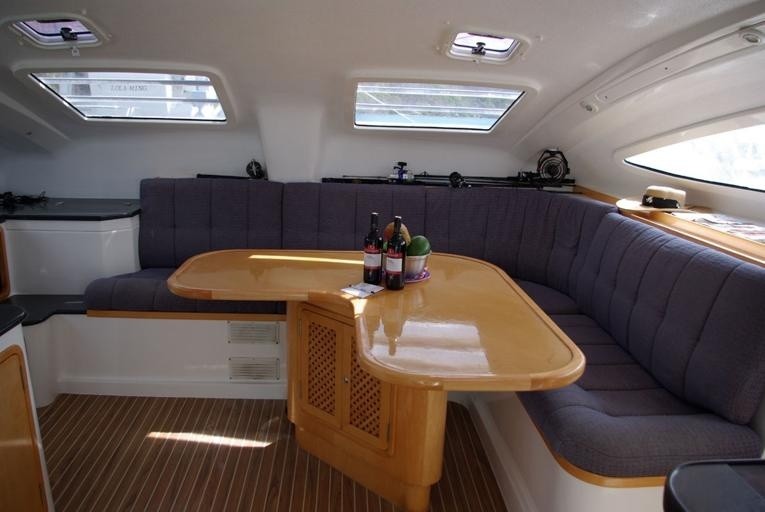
[382,249,432,277]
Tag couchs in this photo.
[82,179,765,512]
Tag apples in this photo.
[383,222,411,250]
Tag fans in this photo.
[536,145,570,187]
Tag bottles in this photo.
[364,212,383,285]
[362,297,382,350]
[383,292,407,355]
[385,216,406,290]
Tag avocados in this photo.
[407,235,431,256]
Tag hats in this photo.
[615,185,713,213]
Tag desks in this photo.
[165,247,586,511]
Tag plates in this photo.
[382,268,431,283]
